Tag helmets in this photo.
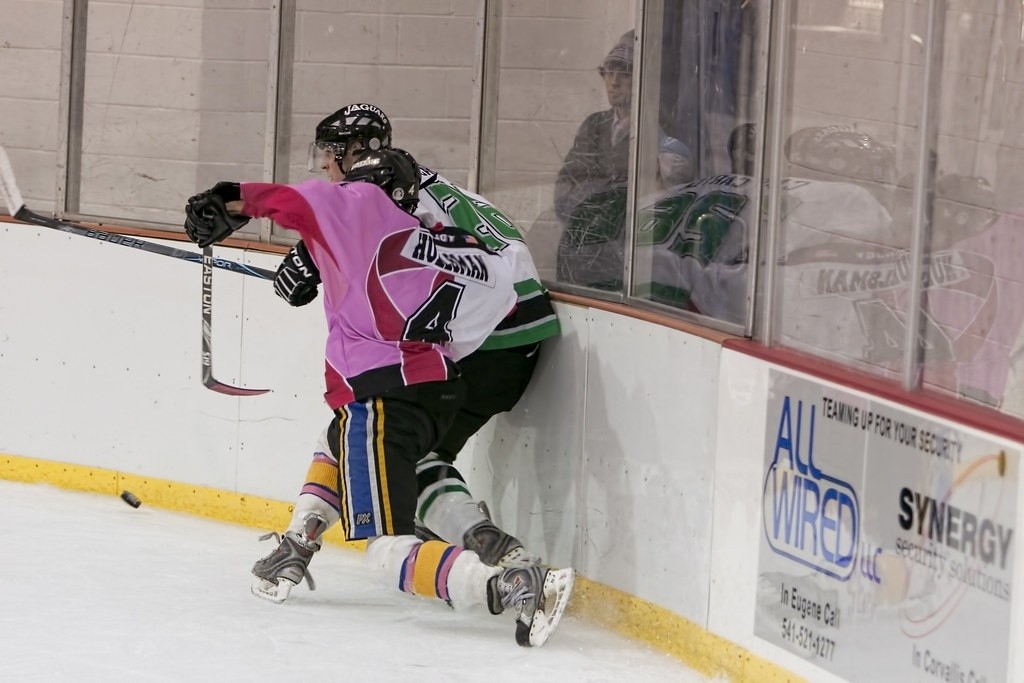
[726,122,761,174]
[933,175,1001,242]
[315,103,393,158]
[342,147,420,203]
[785,127,892,185]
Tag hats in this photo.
[603,29,634,65]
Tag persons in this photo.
[559,123,1010,408]
[553,30,694,316]
[185,102,576,648]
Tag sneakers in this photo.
[415,525,448,544]
[463,500,529,570]
[252,533,322,603]
[486,561,575,647]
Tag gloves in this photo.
[183,182,252,248]
[272,240,322,307]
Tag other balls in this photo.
[120,490,143,509]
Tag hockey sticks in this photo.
[0,145,276,277]
[201,246,276,398]
